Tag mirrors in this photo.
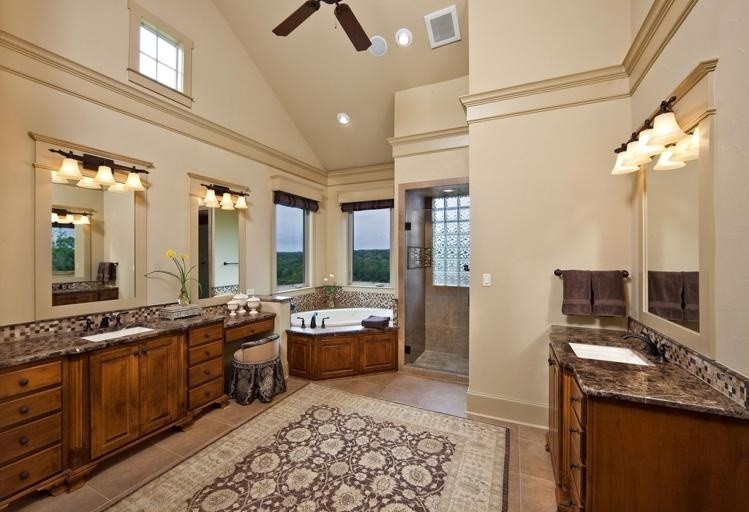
[188,172,251,307]
[52,224,91,283]
[638,64,719,361]
[29,131,153,322]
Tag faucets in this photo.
[310,312,318,328]
[619,332,658,356]
[99,315,114,329]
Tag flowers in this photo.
[144,249,201,304]
[322,274,337,295]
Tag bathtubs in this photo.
[285,307,398,381]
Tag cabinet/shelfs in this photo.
[52,288,118,306]
[545,343,749,512]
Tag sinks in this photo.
[568,340,650,365]
[80,325,156,343]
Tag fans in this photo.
[272,0,372,51]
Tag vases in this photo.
[326,295,335,309]
[177,287,191,306]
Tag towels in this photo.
[97,262,115,282]
[593,271,626,318]
[648,271,698,324]
[562,270,592,316]
[362,315,390,330]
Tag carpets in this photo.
[92,382,510,512]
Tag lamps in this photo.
[610,96,687,175]
[201,184,249,208]
[206,201,235,210]
[48,147,149,191]
[653,126,699,171]
[51,171,129,194]
[52,208,93,225]
